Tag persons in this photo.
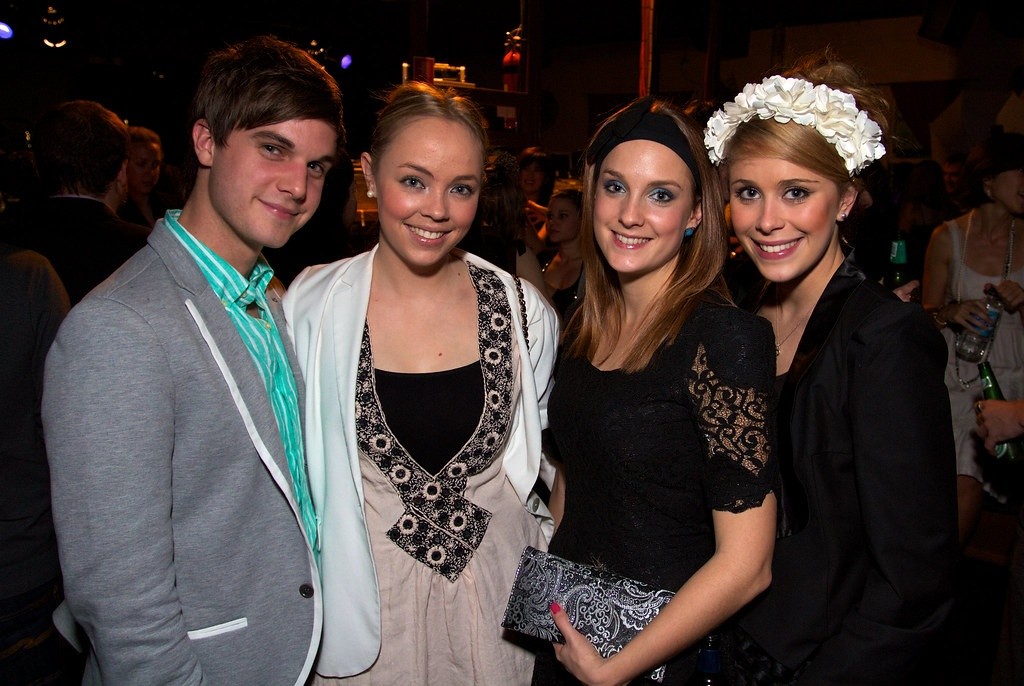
[261,145,767,327]
[840,139,993,305]
[703,44,961,686]
[531,94,779,686]
[972,399,1024,457]
[270,81,561,686]
[0,98,153,309]
[0,249,73,653]
[41,37,347,686]
[116,126,176,228]
[921,132,1024,549]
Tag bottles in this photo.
[883,229,912,293]
[976,360,1024,469]
[955,287,1002,361]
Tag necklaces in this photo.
[952,208,1015,391]
[773,303,813,356]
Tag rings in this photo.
[975,401,983,414]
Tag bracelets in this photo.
[932,305,948,326]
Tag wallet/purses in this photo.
[501,545,676,684]
